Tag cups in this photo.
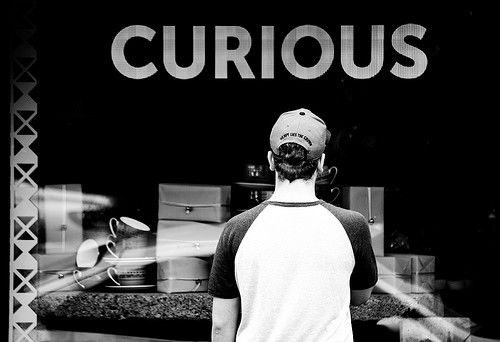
[105,216,150,286]
[74,237,100,268]
[247,190,260,209]
[246,164,263,182]
[314,166,341,205]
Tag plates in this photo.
[104,284,155,291]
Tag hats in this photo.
[269,107,331,161]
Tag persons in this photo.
[207,108,378,342]
